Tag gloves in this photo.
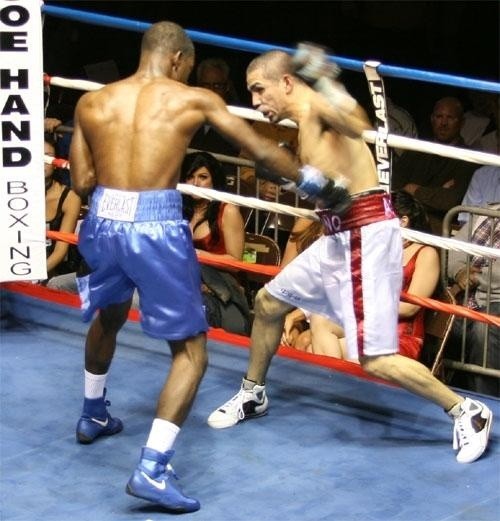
[291,42,331,92]
[297,164,352,217]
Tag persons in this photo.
[41,34,500,396]
[205,40,494,464]
[68,18,352,515]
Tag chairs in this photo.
[424,285,456,384]
[225,177,316,323]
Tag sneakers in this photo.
[208,376,269,429]
[444,397,493,463]
[126,447,199,512]
[76,398,123,443]
